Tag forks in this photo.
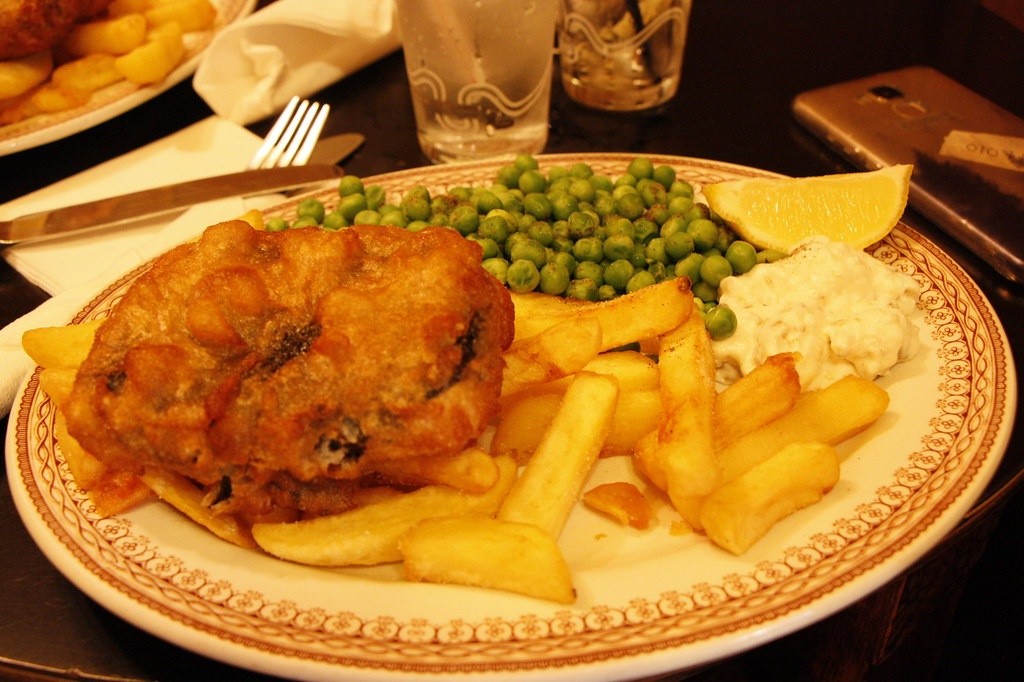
[237,95,330,199]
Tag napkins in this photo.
[192,0,401,127]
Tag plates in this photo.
[5,151,1019,682]
[1,0,259,155]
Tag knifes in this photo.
[292,133,365,162]
[0,162,343,255]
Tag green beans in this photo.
[266,153,756,339]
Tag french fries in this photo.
[31,0,216,111]
[20,278,889,604]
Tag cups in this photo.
[559,0,692,112]
[394,0,557,165]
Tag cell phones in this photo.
[790,69,1023,283]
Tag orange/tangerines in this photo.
[701,164,915,256]
